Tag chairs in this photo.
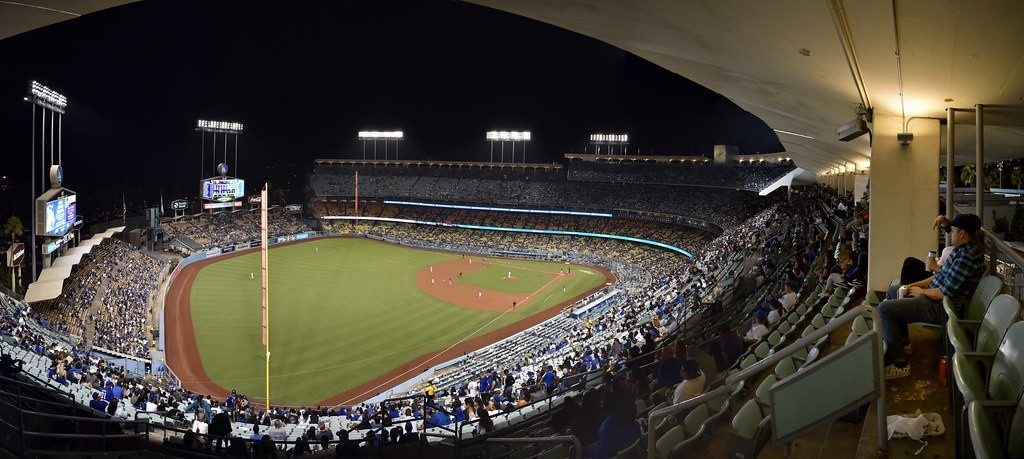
[961,387,1024,459]
[912,260,991,329]
[952,321,1024,459]
[943,277,1003,393]
[0,166,898,459]
[944,294,1021,415]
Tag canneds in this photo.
[925,251,936,272]
[939,356,949,386]
[899,286,908,299]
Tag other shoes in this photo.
[903,341,914,356]
[817,291,831,297]
[884,360,911,380]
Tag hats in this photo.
[752,309,767,320]
[942,212,983,237]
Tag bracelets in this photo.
[943,224,952,234]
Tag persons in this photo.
[161,207,312,258]
[0,295,558,459]
[558,177,870,459]
[310,162,797,262]
[250,272,254,279]
[430,261,511,300]
[876,213,985,381]
[48,239,166,359]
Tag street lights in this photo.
[486,131,531,164]
[196,119,243,181]
[359,130,404,161]
[24,80,67,284]
[589,134,629,156]
